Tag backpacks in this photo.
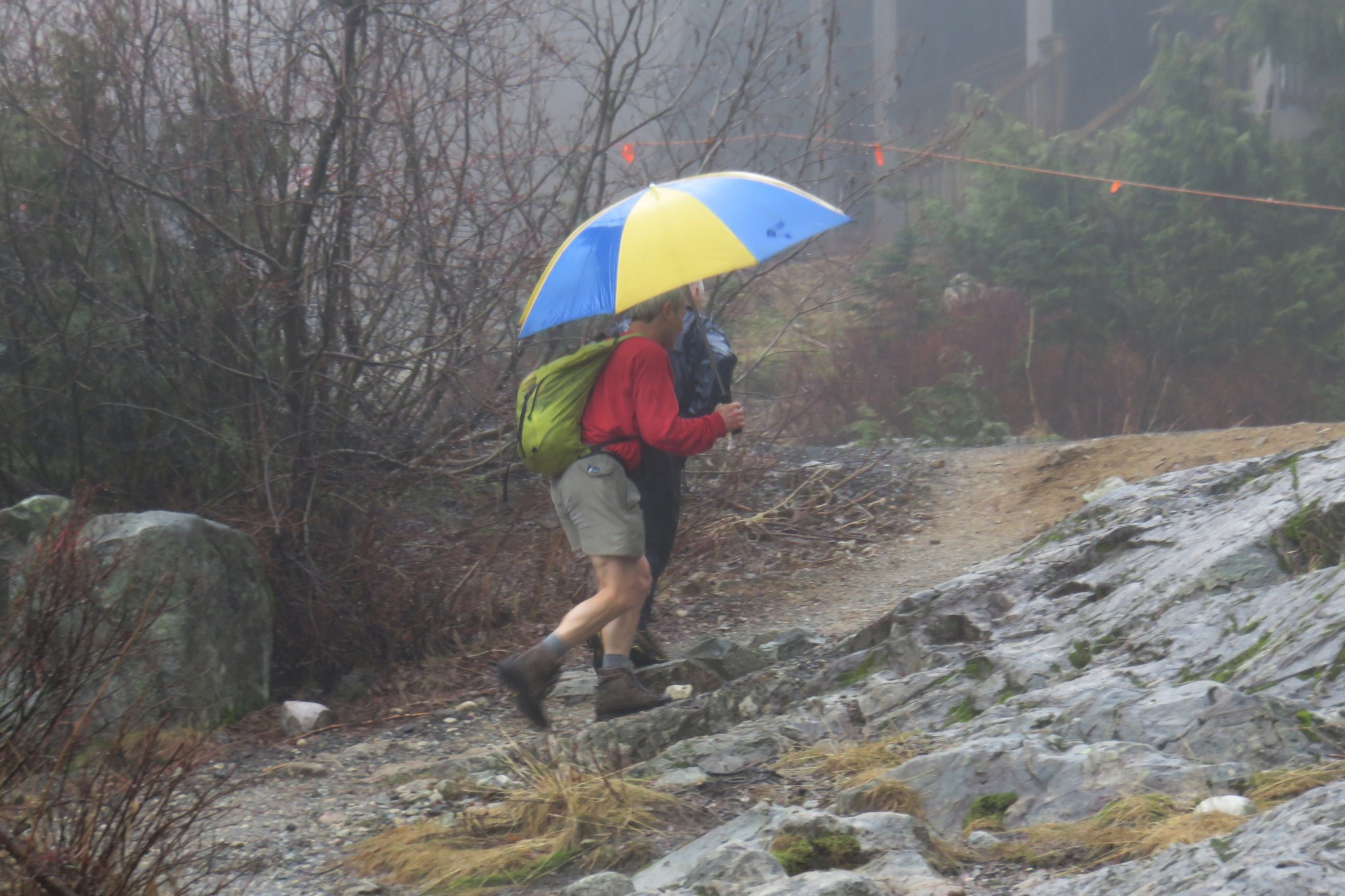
[677,313,738,419]
[515,332,673,480]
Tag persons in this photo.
[587,281,737,676]
[497,289,747,733]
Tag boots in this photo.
[594,664,673,722]
[499,641,563,729]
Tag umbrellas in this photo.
[515,169,856,434]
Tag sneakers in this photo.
[582,630,605,668]
[630,629,672,666]
